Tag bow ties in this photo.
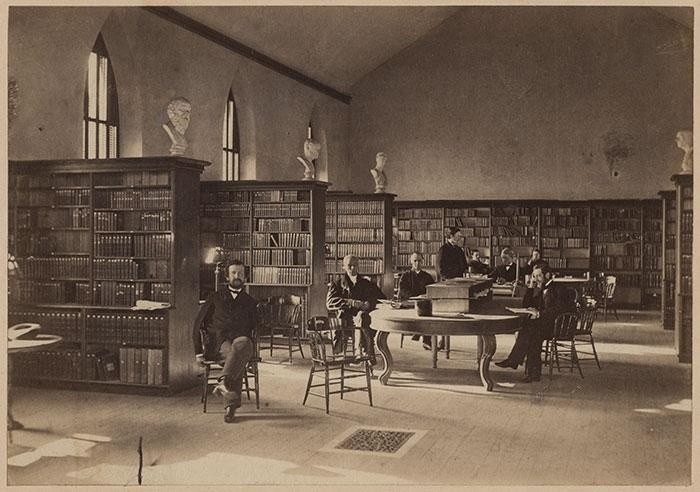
[229,290,240,294]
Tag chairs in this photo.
[195,329,261,414]
[303,316,373,415]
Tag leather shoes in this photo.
[212,382,238,400]
[495,359,518,369]
[224,407,235,422]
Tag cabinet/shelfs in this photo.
[8,156,397,397]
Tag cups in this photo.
[418,300,433,315]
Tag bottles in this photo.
[463,268,469,278]
[468,267,471,278]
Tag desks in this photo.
[369,298,540,392]
[7,333,61,430]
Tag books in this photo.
[590,207,641,310]
[541,208,590,279]
[325,201,383,290]
[492,208,539,267]
[444,208,489,277]
[199,190,312,339]
[392,208,442,292]
[8,171,172,386]
[641,184,692,363]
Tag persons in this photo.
[326,255,388,368]
[438,227,524,285]
[194,258,260,424]
[673,130,693,170]
[495,249,570,384]
[163,97,193,147]
[398,251,440,352]
[371,152,388,185]
[297,139,321,172]
